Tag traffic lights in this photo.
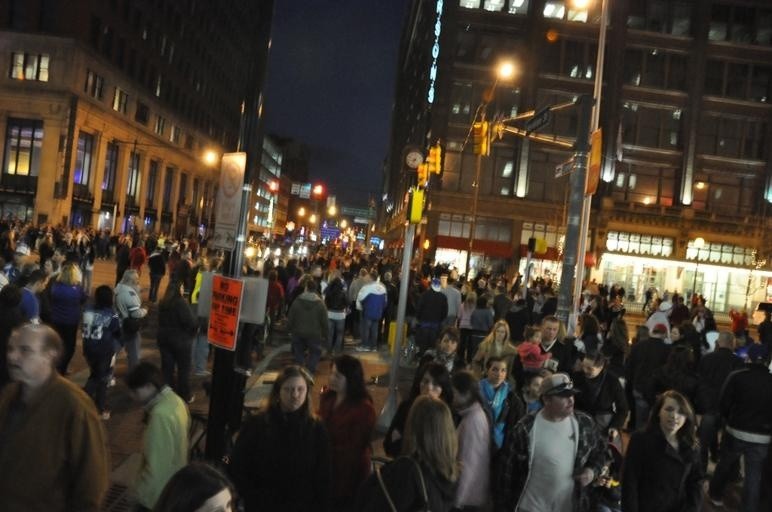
[473,120,488,158]
[405,189,425,225]
[527,237,536,252]
[428,144,442,175]
[417,161,431,189]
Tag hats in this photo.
[431,279,441,292]
[660,302,672,311]
[16,243,30,255]
[543,374,581,397]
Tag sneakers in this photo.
[356,346,370,351]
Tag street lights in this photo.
[125,138,219,238]
[463,54,522,282]
[554,158,576,178]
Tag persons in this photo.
[0,215,772,512]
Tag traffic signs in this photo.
[524,104,552,136]
